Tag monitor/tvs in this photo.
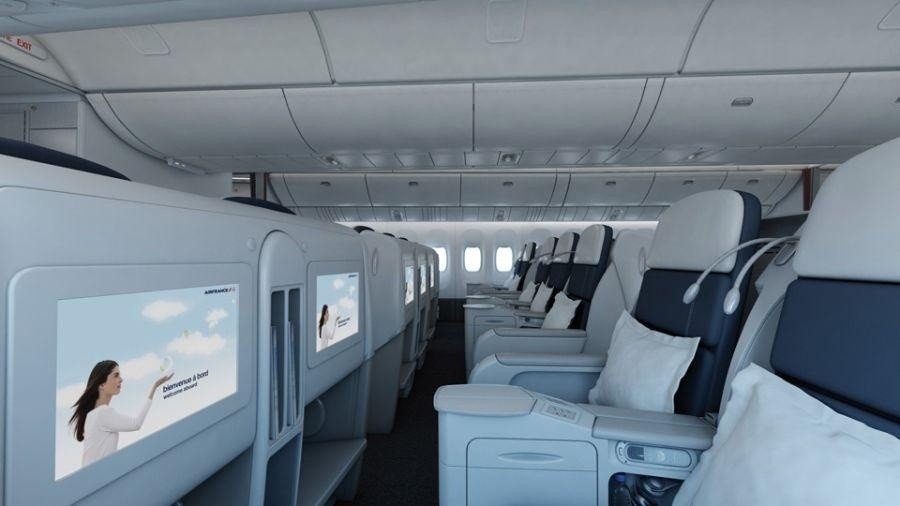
[305,259,365,368]
[404,260,435,311]
[4,263,254,504]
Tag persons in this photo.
[317,303,342,350]
[67,358,175,469]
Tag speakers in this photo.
[498,151,521,167]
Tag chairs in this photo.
[4,143,445,503]
[433,139,893,499]
